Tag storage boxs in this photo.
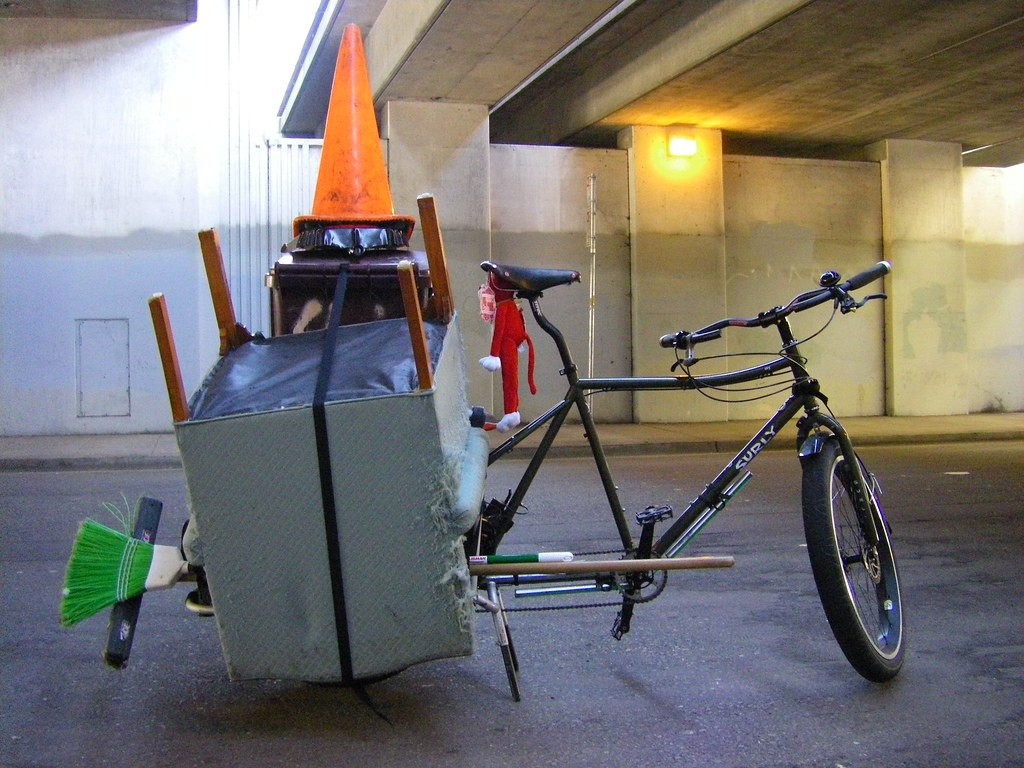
[268,252,432,337]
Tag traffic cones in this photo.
[290,18,420,240]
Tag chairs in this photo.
[149,195,494,684]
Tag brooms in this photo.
[56,493,735,670]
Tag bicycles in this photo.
[480,259,907,688]
